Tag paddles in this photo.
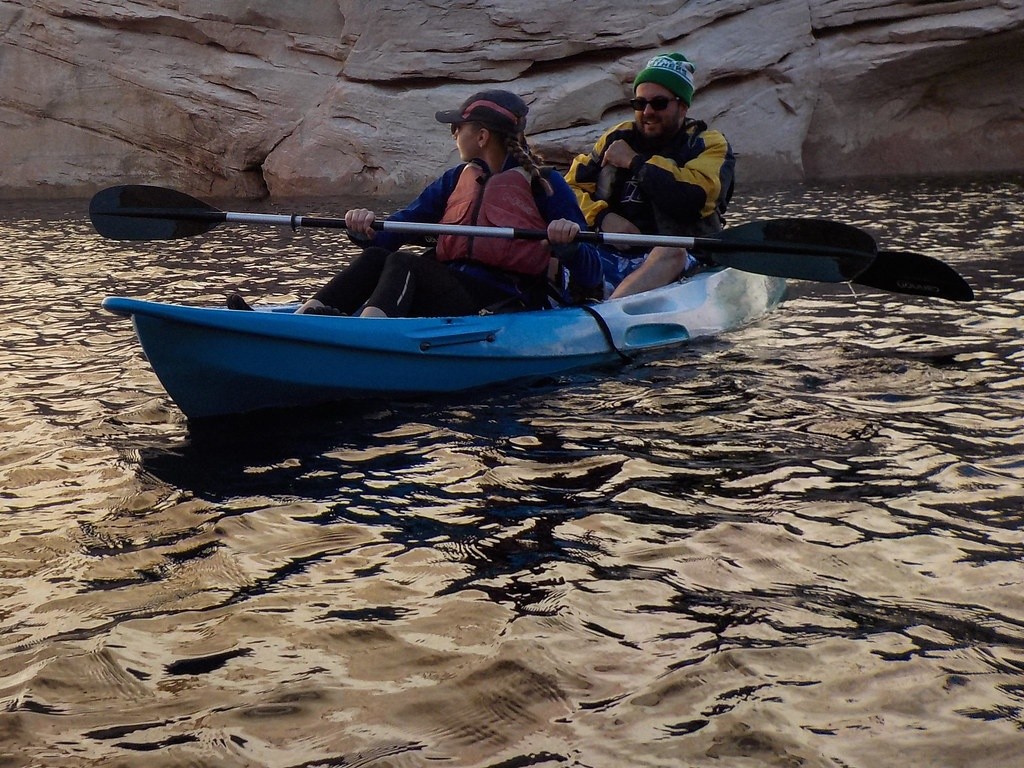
[88,182,875,284]
[868,247,976,303]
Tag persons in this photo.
[549,53,736,300]
[226,88,604,319]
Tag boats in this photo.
[102,269,788,419]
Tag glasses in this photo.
[629,94,680,111]
[450,119,491,135]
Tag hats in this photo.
[632,52,697,109]
[435,90,529,136]
